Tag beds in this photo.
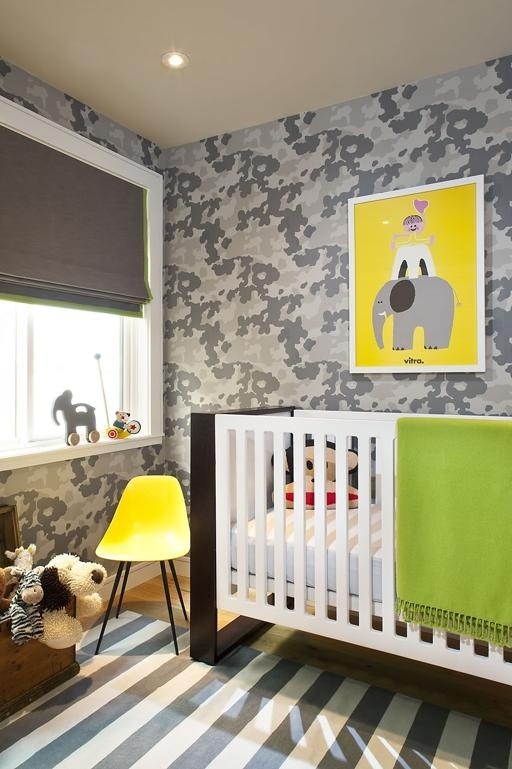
[188,407,511,687]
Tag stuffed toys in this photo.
[0,544,107,650]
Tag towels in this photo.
[394,415,511,648]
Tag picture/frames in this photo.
[347,174,487,377]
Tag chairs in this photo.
[93,471,189,656]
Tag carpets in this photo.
[1,611,511,767]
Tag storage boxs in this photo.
[1,503,81,720]
